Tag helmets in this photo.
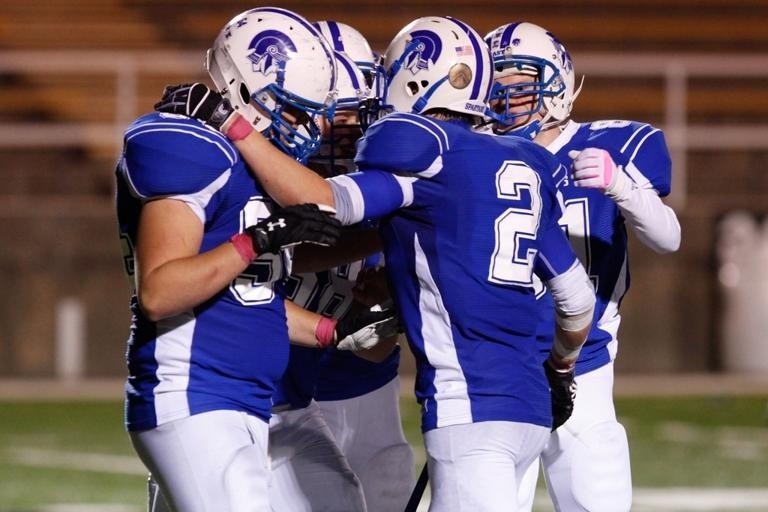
[483,21,577,128]
[204,5,338,159]
[309,14,494,162]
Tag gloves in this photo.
[540,351,579,432]
[567,146,619,192]
[332,293,404,354]
[231,202,345,265]
[151,78,237,133]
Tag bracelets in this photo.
[230,231,260,268]
[223,116,256,142]
[312,315,339,347]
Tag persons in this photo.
[152,14,598,512]
[474,22,684,512]
[116,7,397,510]
[269,17,416,511]
